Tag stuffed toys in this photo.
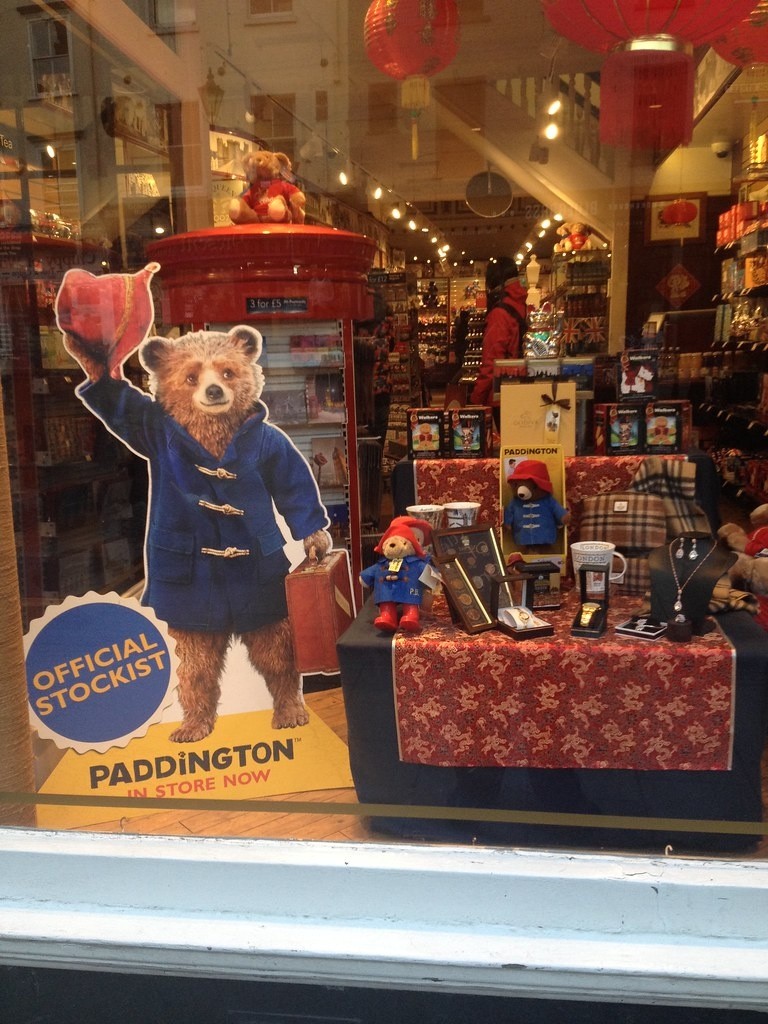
[359,515,432,630]
[505,460,571,554]
[229,151,306,224]
[554,223,592,250]
[61,325,331,744]
[718,505,768,590]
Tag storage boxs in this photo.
[591,401,647,454]
[285,546,359,675]
[448,405,494,458]
[406,406,451,460]
[646,400,694,454]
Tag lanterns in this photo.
[541,0,761,150]
[712,0,768,76]
[662,199,697,244]
[364,0,459,160]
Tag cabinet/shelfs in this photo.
[0,191,768,851]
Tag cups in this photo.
[443,502,481,528]
[406,505,444,530]
[570,541,627,591]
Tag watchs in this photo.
[580,602,599,626]
[515,607,530,627]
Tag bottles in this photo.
[656,346,746,402]
[566,285,608,317]
[567,255,608,283]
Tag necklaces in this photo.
[669,537,717,612]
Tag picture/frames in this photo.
[433,524,514,626]
[645,193,707,246]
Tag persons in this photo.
[649,531,738,621]
[452,311,469,363]
[357,281,395,350]
[469,256,528,405]
